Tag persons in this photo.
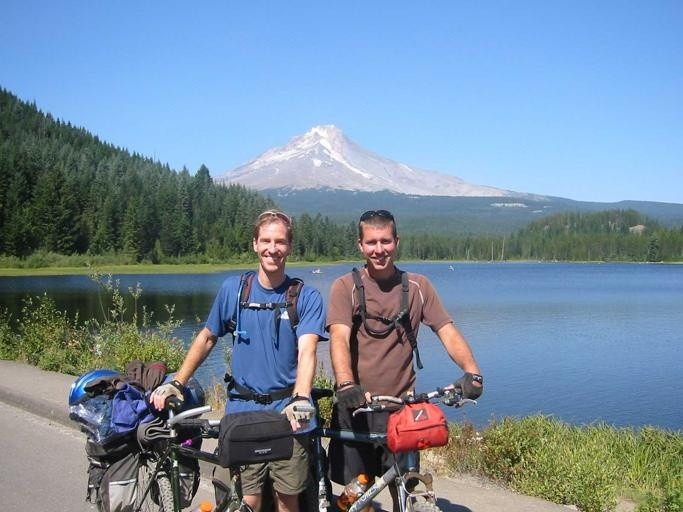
[325,208,484,511]
[148,205,330,512]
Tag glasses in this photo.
[359,210,395,224]
[257,211,292,227]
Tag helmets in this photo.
[67,369,119,406]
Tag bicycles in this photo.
[304,386,479,512]
[93,391,318,512]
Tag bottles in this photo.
[335,473,369,511]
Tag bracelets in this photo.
[285,393,312,407]
[166,380,185,394]
[335,379,355,391]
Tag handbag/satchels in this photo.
[386,401,449,453]
[216,409,293,467]
[83,417,202,512]
